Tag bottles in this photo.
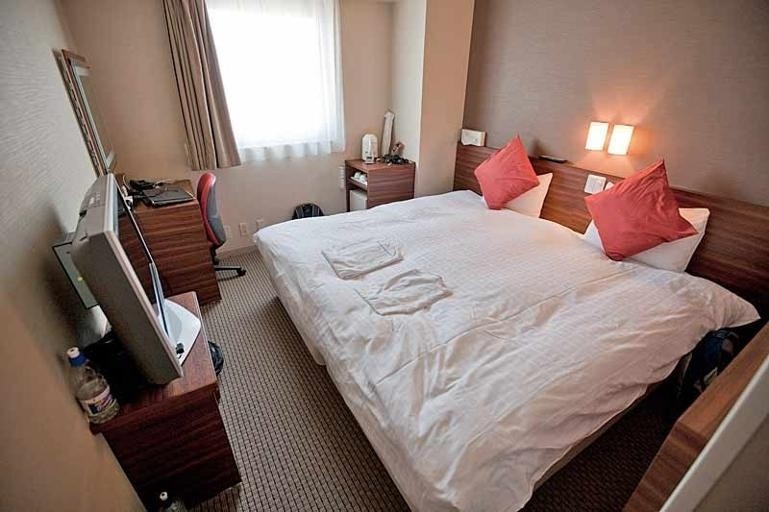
[159,491,189,512]
[67,348,121,426]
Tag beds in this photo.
[256,139,769,511]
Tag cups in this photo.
[354,172,367,182]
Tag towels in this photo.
[355,265,452,316]
[319,233,404,280]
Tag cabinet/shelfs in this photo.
[92,294,241,510]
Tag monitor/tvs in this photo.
[71,173,202,385]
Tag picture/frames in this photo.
[53,41,116,177]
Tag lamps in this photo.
[585,121,636,155]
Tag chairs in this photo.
[195,169,247,281]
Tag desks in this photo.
[98,180,221,305]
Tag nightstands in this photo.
[344,156,416,210]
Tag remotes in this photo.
[539,154,568,163]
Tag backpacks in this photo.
[292,203,323,220]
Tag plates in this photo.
[351,176,367,185]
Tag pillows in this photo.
[583,157,697,261]
[478,170,552,219]
[473,132,540,209]
[582,203,709,274]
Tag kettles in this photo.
[360,133,379,164]
